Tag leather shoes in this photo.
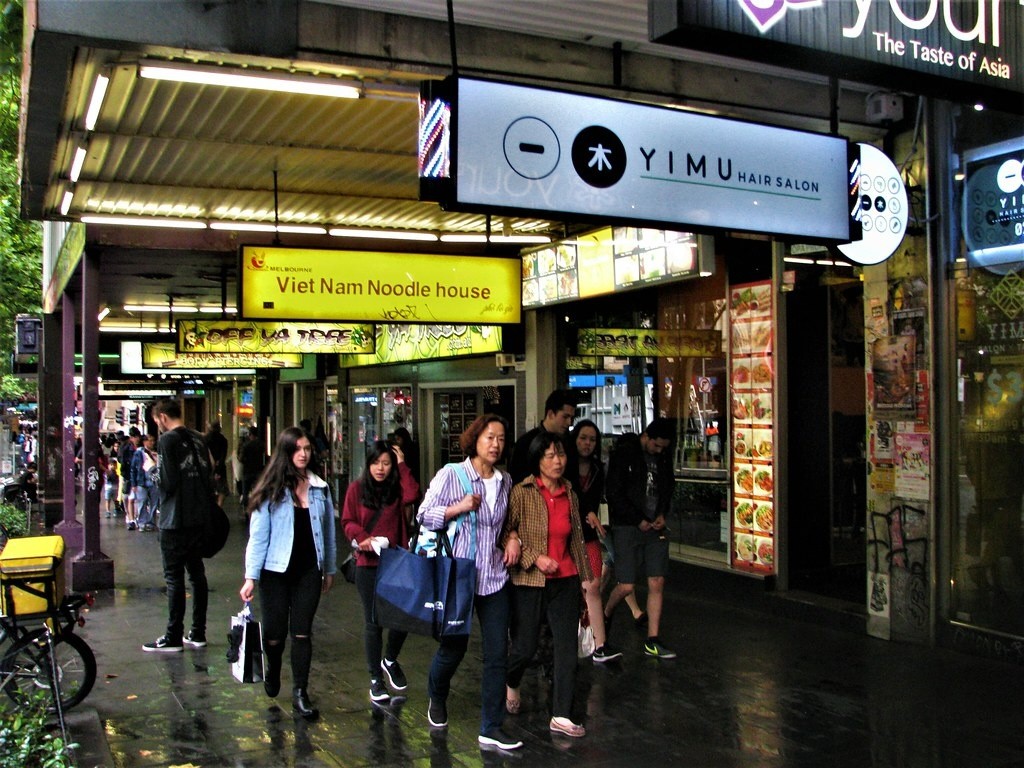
[292,690,319,722]
[265,668,280,697]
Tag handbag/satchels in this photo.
[197,500,230,558]
[226,601,264,683]
[340,552,356,583]
[372,519,475,638]
[578,578,605,658]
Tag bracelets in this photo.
[506,536,522,546]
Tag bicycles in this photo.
[0,588,98,716]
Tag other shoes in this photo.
[144,523,153,532]
[550,719,585,737]
[603,614,612,630]
[127,522,135,530]
[505,697,520,713]
[631,611,648,628]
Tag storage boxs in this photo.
[0,536,67,626]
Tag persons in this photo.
[392,427,419,529]
[415,415,523,750]
[74,420,162,532]
[12,418,39,502]
[204,418,327,520]
[506,388,678,662]
[239,427,337,716]
[340,440,420,701]
[142,398,215,653]
[499,431,593,738]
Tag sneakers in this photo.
[427,697,448,726]
[391,695,406,705]
[593,645,624,663]
[644,642,676,658]
[183,630,207,647]
[381,656,408,690]
[369,684,389,701]
[478,729,523,750]
[141,635,183,652]
[371,701,385,711]
